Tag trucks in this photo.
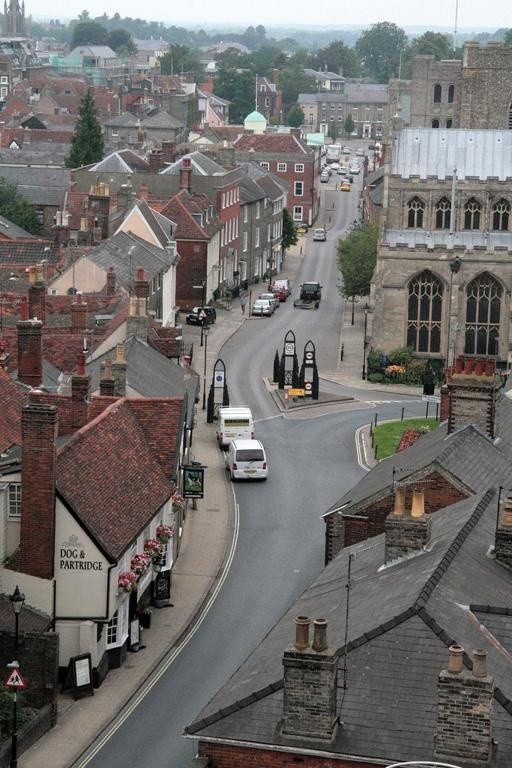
[215,407,254,450]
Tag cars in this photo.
[271,285,287,302]
[251,300,275,317]
[319,142,381,192]
[258,292,281,309]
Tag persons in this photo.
[240,295,247,314]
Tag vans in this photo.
[222,439,269,482]
[313,228,328,241]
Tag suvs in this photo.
[299,280,323,304]
[272,277,293,296]
[185,305,218,327]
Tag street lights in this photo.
[201,323,209,411]
[360,300,370,381]
[8,583,27,768]
[267,236,274,292]
[201,275,209,311]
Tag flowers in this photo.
[114,490,189,596]
